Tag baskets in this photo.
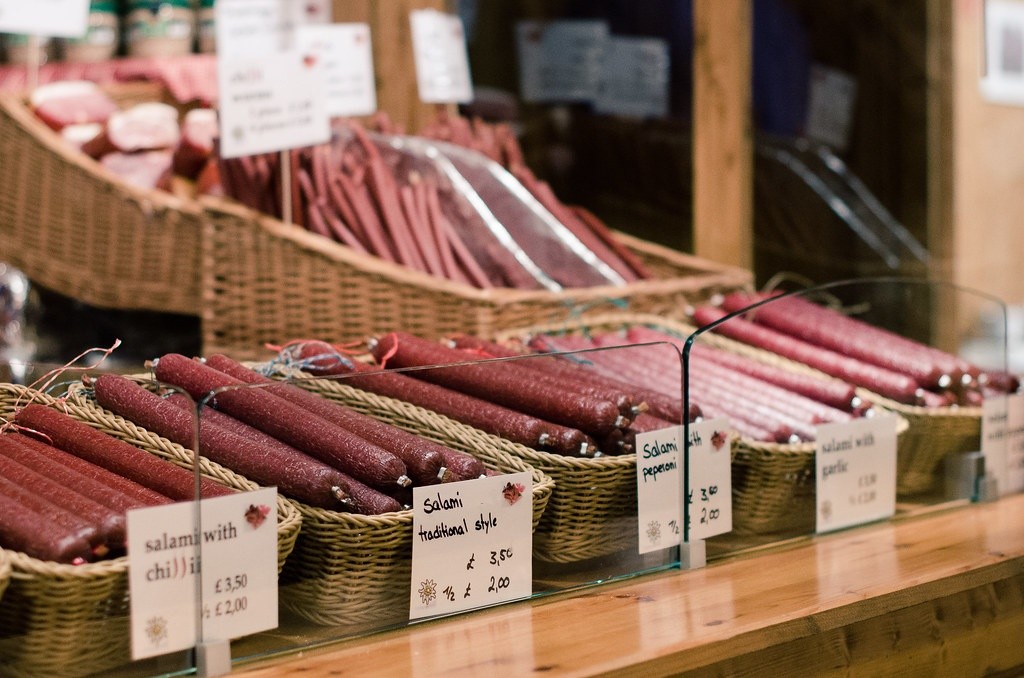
[0,67,985,677]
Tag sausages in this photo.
[0,114,1020,566]
